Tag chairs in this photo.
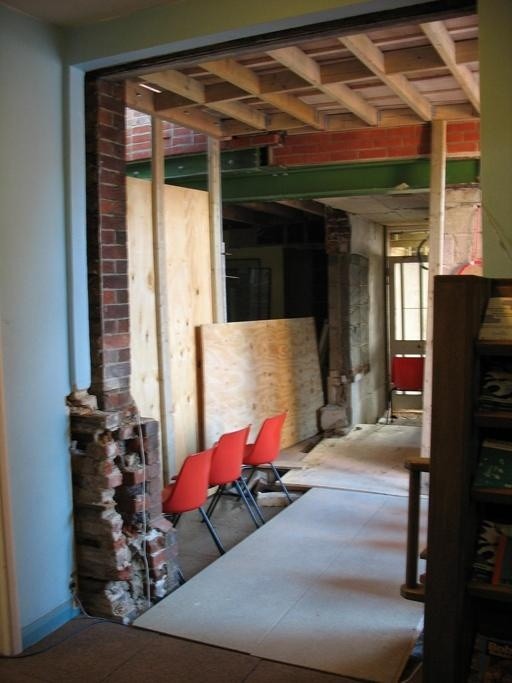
[159,408,295,556]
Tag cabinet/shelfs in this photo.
[422,273,511,682]
